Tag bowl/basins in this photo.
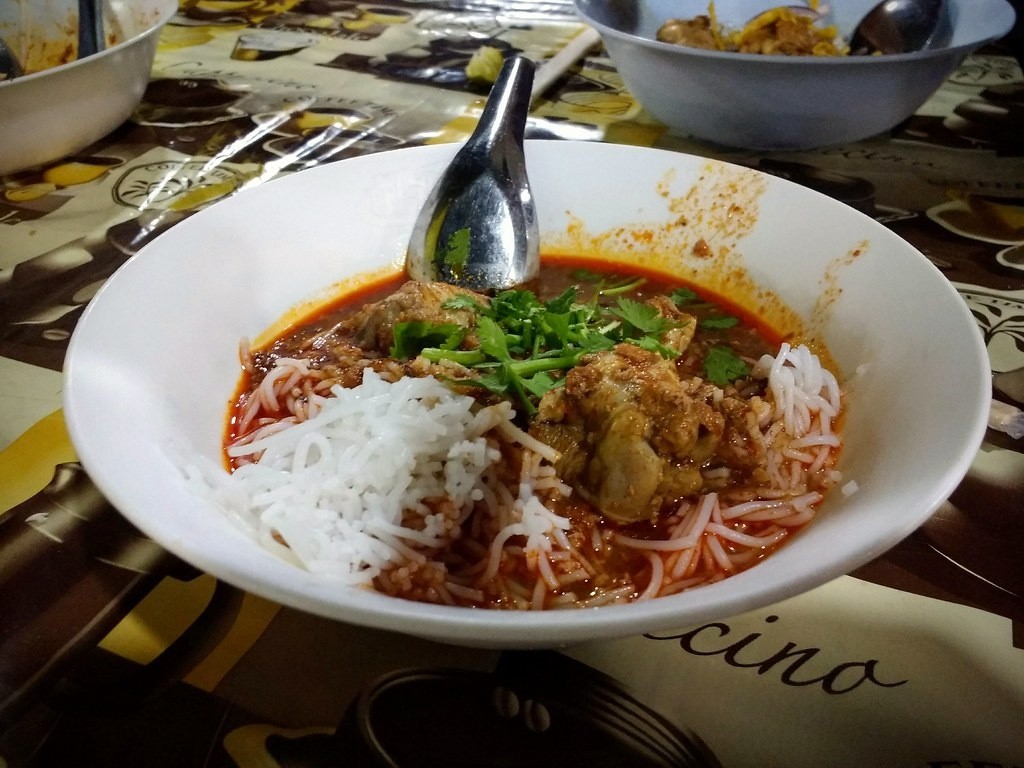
[571,0,1016,151]
[0,0,179,176]
[60,135,991,650]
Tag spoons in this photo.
[845,0,948,55]
[407,56,540,296]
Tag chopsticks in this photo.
[532,26,601,102]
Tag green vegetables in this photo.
[385,226,751,421]
